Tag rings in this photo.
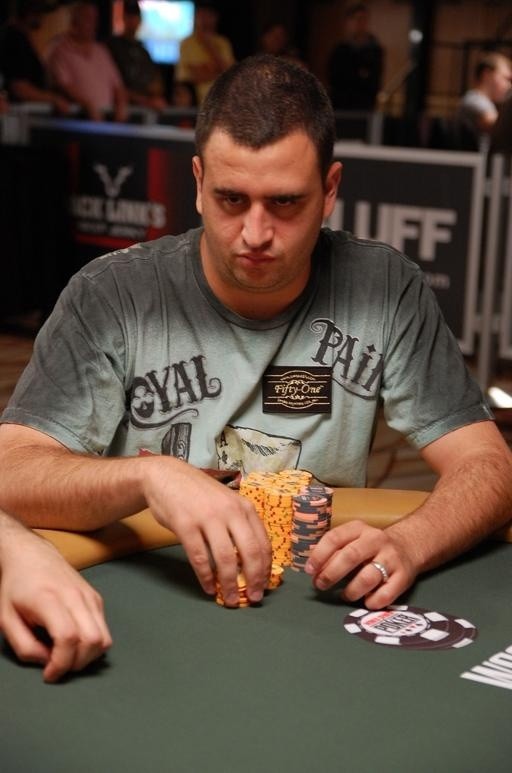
[369,560,389,584]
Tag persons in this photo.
[450,54,512,151]
[0,0,384,125]
[0,54,511,613]
[0,512,115,684]
